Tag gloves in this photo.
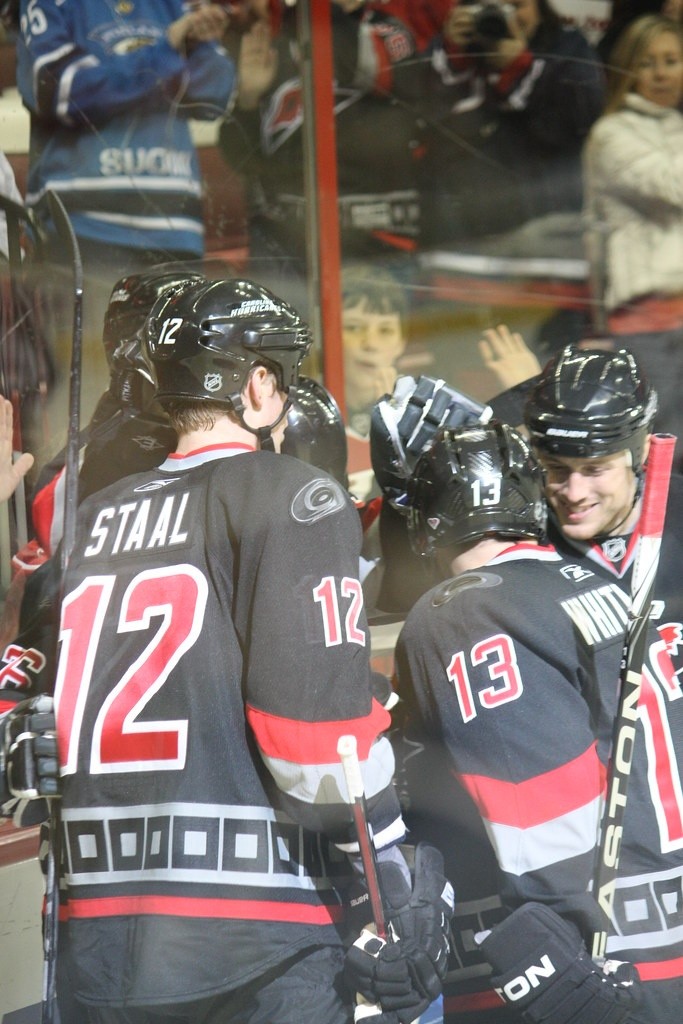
[0,695,62,827]
[472,902,645,1024]
[344,843,454,1023]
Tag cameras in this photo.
[474,3,513,40]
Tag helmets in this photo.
[145,279,314,405]
[103,271,205,374]
[405,422,547,558]
[524,342,659,468]
[281,376,349,491]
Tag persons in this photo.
[0,0,683,1024]
